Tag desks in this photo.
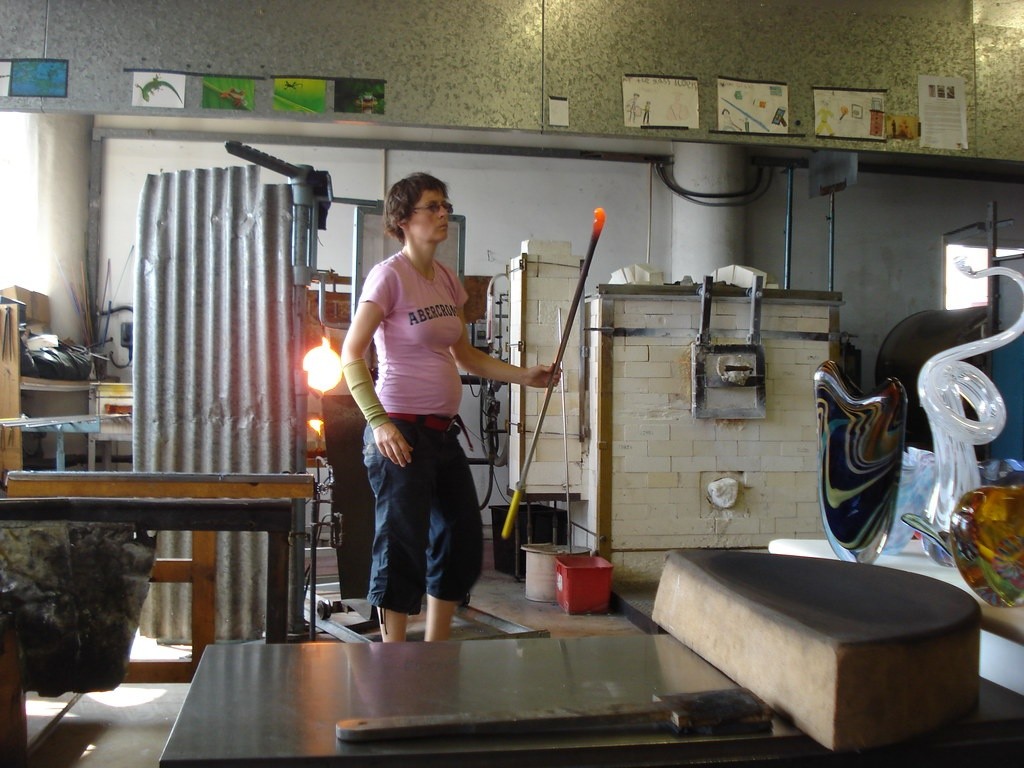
[768,538,1024,698]
[85,383,134,471]
[0,469,315,766]
[159,635,1024,768]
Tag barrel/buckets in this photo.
[520,543,593,602]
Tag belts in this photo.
[387,413,475,452]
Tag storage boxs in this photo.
[555,556,614,614]
[27,292,49,323]
[25,320,51,336]
[0,286,32,324]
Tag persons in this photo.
[340,171,564,641]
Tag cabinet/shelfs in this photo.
[0,305,101,489]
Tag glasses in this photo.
[410,200,454,215]
